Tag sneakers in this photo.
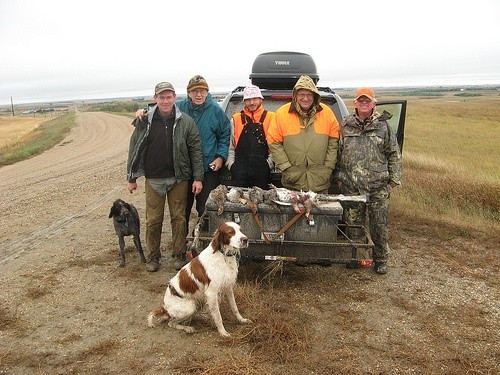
[174,254,186,269]
[146,256,159,271]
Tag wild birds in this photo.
[208,182,317,218]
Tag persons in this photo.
[335,87,401,274]
[136,75,231,234]
[225,85,275,190]
[267,75,340,194]
[126,82,204,271]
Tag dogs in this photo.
[147,221,254,337]
[109,198,147,267]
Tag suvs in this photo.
[219,51,407,225]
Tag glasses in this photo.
[357,98,372,103]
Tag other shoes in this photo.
[375,262,387,273]
[347,264,359,269]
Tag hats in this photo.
[155,82,175,94]
[187,75,209,91]
[355,87,375,101]
[243,84,264,102]
